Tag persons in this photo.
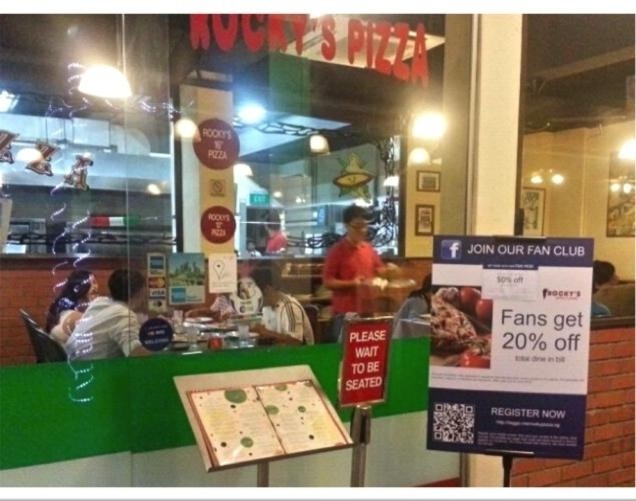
[590,262,611,315]
[322,202,402,343]
[42,269,94,347]
[596,259,620,286]
[249,212,288,257]
[181,265,264,321]
[61,268,175,360]
[392,270,435,336]
[245,268,314,349]
[85,270,99,302]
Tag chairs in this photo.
[17,306,68,363]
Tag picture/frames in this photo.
[521,186,546,237]
[416,169,441,192]
[414,204,435,237]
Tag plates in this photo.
[182,317,261,342]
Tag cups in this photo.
[173,310,184,334]
[186,326,198,348]
[237,325,250,349]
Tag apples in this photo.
[207,337,223,350]
[460,344,491,368]
[460,287,493,321]
